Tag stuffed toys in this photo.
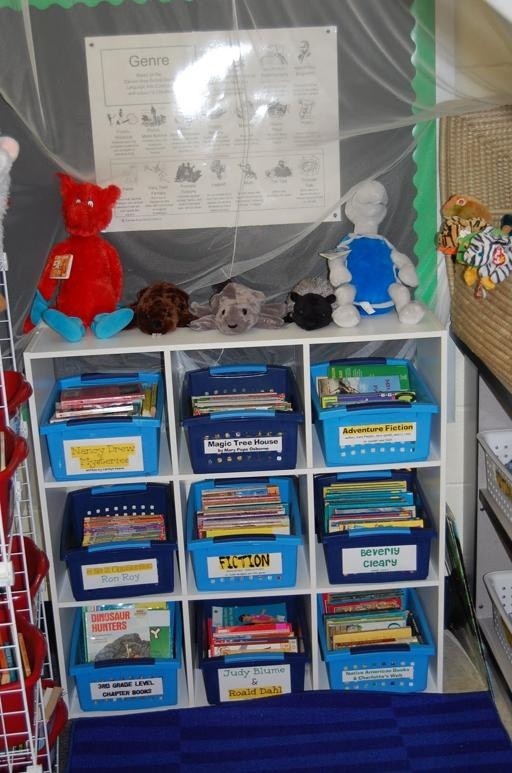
[320,178,426,327]
[456,225,512,300]
[188,282,288,335]
[121,279,197,336]
[0,136,21,270]
[283,274,336,331]
[22,172,133,342]
[437,193,493,255]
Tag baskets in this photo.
[314,586,439,697]
[307,355,442,467]
[182,474,308,593]
[56,480,179,601]
[177,361,305,475]
[65,600,184,712]
[474,428,512,666]
[439,88,512,395]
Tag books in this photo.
[2,640,16,682]
[0,646,12,686]
[35,687,53,748]
[195,486,290,582]
[49,383,157,424]
[315,366,417,456]
[321,588,423,652]
[16,632,31,676]
[44,684,63,743]
[82,602,174,662]
[1,429,7,472]
[323,481,423,534]
[82,515,167,546]
[202,602,297,658]
[192,392,293,464]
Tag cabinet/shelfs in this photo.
[471,353,512,702]
[1,258,56,773]
[22,313,454,720]
